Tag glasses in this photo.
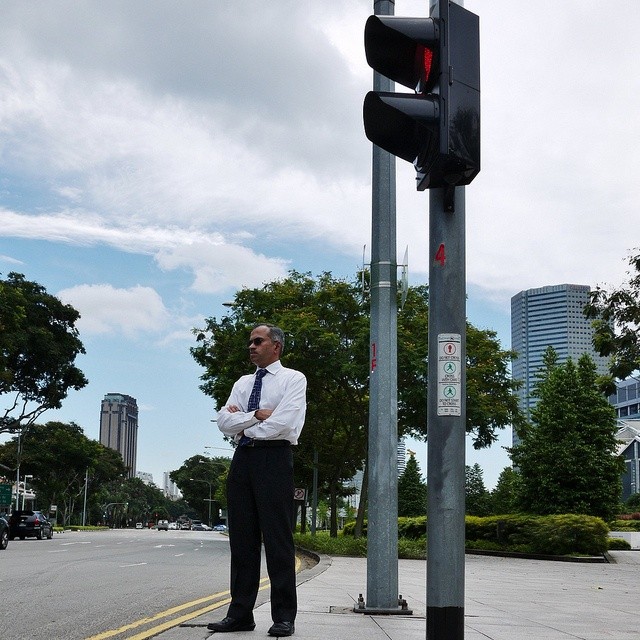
[247,338,274,347]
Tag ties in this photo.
[238,369,269,447]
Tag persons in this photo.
[206,321,310,638]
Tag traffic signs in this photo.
[0,484,12,505]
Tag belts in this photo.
[247,440,290,447]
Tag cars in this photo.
[135,516,212,531]
[0,508,11,550]
[7,510,54,541]
[214,524,229,532]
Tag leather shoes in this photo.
[208,617,255,632]
[268,621,294,637]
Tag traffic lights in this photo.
[362,0,481,214]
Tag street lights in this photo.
[199,460,225,466]
[15,414,31,511]
[204,446,235,452]
[190,478,212,527]
[210,417,220,423]
